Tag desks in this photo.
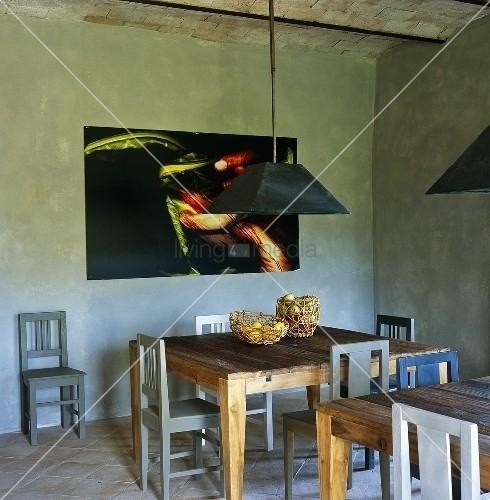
[129,324,449,500]
[317,373,490,500]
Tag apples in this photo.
[278,293,318,333]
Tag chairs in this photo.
[19,311,87,445]
[340,314,414,470]
[281,338,389,499]
[195,313,274,454]
[390,350,482,500]
[138,333,225,498]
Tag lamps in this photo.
[202,0,350,215]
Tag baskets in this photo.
[229,293,321,346]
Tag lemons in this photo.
[234,320,285,342]
